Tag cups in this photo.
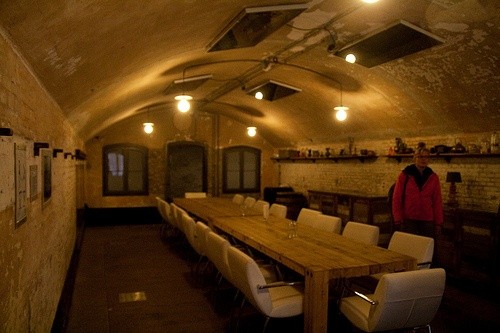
[480,133,498,153]
[289,221,296,238]
[241,204,246,216]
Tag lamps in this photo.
[175,68,192,113]
[247,113,257,137]
[447,172,462,207]
[143,108,154,134]
[334,82,349,122]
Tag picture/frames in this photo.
[41,150,53,209]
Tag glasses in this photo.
[418,155,429,159]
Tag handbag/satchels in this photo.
[387,171,408,214]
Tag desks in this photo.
[215,215,418,333]
[173,197,273,230]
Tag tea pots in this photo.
[451,137,465,153]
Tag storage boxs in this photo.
[279,150,299,158]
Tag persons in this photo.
[391,147,443,239]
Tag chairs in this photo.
[154,192,446,332]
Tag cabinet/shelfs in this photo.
[308,189,394,248]
[443,202,500,298]
